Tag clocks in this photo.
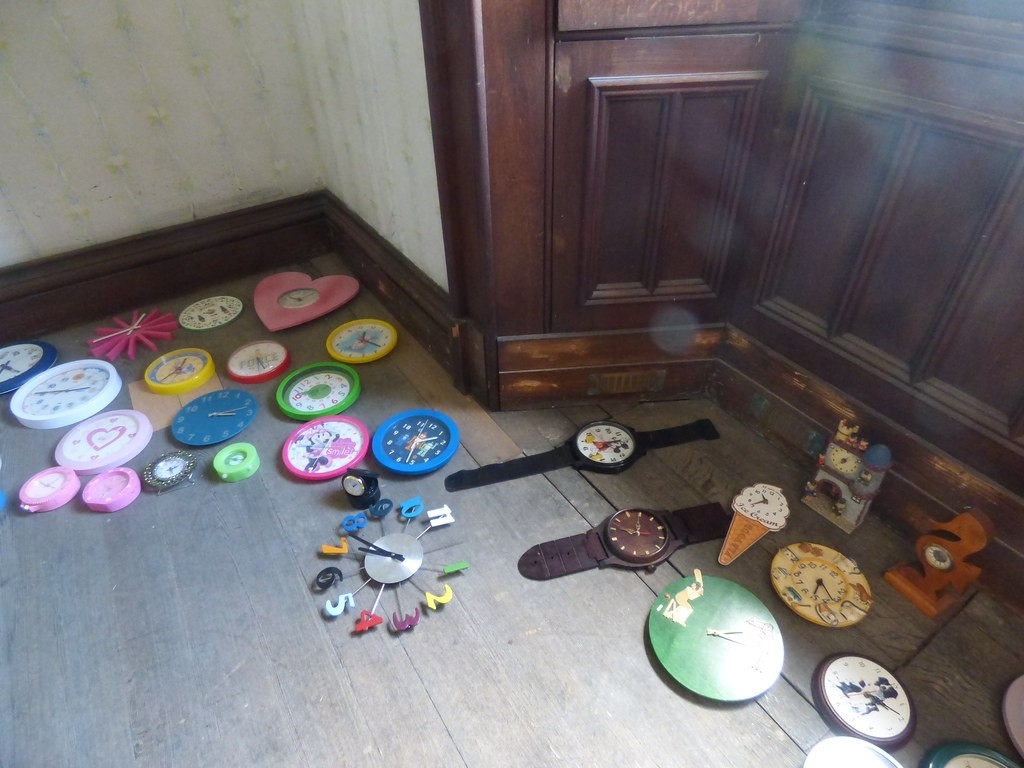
[371,409,460,477]
[54,409,153,475]
[282,416,370,481]
[326,319,398,364]
[213,442,261,483]
[649,419,1024,768]
[10,358,122,430]
[87,308,178,362]
[143,451,197,496]
[253,272,360,332]
[316,497,471,632]
[18,466,81,512]
[0,340,59,395]
[145,347,216,395]
[341,468,381,510]
[275,361,362,421]
[224,339,292,384]
[82,466,141,512]
[171,390,258,445]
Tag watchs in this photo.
[444,418,720,493]
[518,502,731,582]
